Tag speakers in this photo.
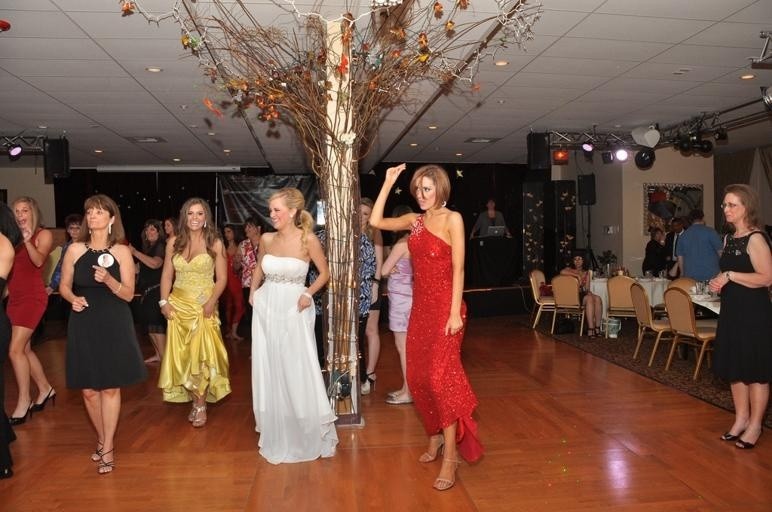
[579,175,595,205]
[527,132,551,170]
[43,139,70,177]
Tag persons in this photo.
[48,214,86,294]
[247,187,340,462]
[310,197,415,402]
[709,185,772,450]
[470,199,510,242]
[130,218,177,364]
[663,218,690,279]
[158,197,231,428]
[642,227,668,277]
[60,194,137,475]
[561,251,603,339]
[369,161,473,490]
[10,195,58,424]
[222,218,265,340]
[672,207,723,282]
[0,205,20,477]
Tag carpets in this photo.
[513,313,772,432]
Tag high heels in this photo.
[7,401,32,424]
[419,434,444,462]
[435,456,458,490]
[361,370,376,395]
[32,385,56,411]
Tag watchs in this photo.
[726,269,733,283]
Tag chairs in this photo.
[606,276,637,339]
[527,268,585,336]
[629,282,684,366]
[662,287,719,380]
[666,277,700,297]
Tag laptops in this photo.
[488,226,504,237]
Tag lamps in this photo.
[7,142,22,157]
[552,113,727,170]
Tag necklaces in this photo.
[424,207,442,218]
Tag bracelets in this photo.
[303,292,313,300]
[158,299,168,307]
[114,282,121,295]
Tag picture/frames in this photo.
[643,183,705,236]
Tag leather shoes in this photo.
[386,390,413,404]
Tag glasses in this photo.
[720,203,737,209]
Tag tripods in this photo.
[579,205,599,271]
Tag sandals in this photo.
[720,422,764,449]
[92,440,114,473]
[588,327,602,339]
[189,404,207,427]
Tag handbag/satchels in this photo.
[540,285,553,296]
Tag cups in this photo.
[603,263,630,279]
[694,281,718,300]
[646,269,667,282]
